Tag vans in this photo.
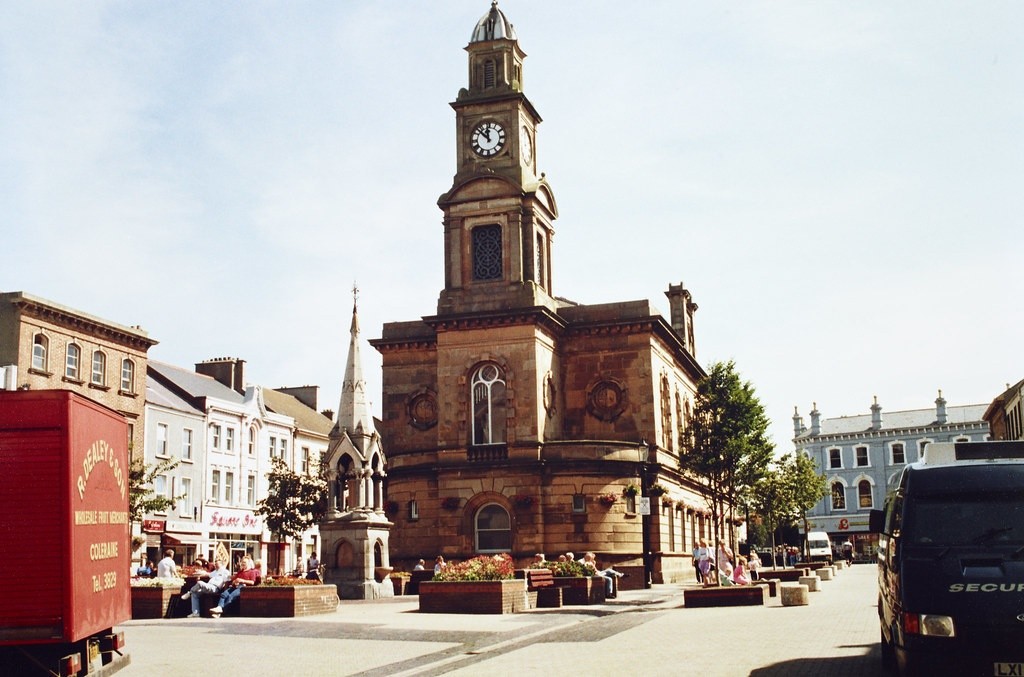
[803,533,835,566]
[867,440,1024,677]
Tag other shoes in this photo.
[187,610,200,618]
[621,574,631,580]
[209,606,223,613]
[212,614,221,618]
[181,591,192,600]
[606,594,615,599]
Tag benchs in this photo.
[176,575,260,612]
[528,570,571,606]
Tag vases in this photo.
[677,507,680,510]
[448,504,455,510]
[520,502,530,507]
[543,576,607,605]
[239,584,336,617]
[419,579,527,614]
[687,511,691,514]
[391,507,397,513]
[663,502,667,507]
[131,588,181,618]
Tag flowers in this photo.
[600,494,617,506]
[517,493,534,502]
[527,561,596,577]
[663,497,742,527]
[442,496,460,508]
[383,502,398,518]
[432,554,514,579]
[130,576,183,588]
[264,577,322,586]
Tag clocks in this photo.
[466,116,511,160]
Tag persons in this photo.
[296,556,303,578]
[531,553,547,566]
[434,555,447,572]
[577,552,632,600]
[693,537,762,588]
[181,559,231,618]
[307,552,320,577]
[137,547,263,580]
[774,542,803,567]
[830,541,856,568]
[210,554,256,618]
[558,552,575,563]
[413,559,425,570]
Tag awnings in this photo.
[164,533,219,545]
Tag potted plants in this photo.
[649,485,668,497]
[624,485,638,497]
